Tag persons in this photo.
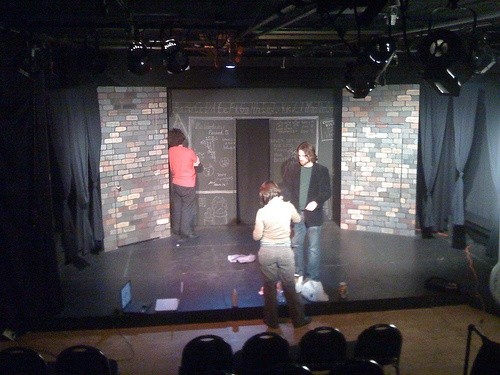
[279,141,331,284]
[168,128,201,240]
[252,180,312,328]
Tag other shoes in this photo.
[173,230,199,239]
[294,317,312,329]
[264,319,279,329]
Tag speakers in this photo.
[452,225,468,250]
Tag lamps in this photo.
[345,1,497,99]
[126,13,255,74]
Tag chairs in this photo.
[0,343,120,375]
[180,323,401,375]
[460,323,500,375]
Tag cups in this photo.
[338,281,348,297]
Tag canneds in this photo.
[338,281,349,297]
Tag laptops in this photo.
[120,281,149,313]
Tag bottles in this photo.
[231,288,239,307]
